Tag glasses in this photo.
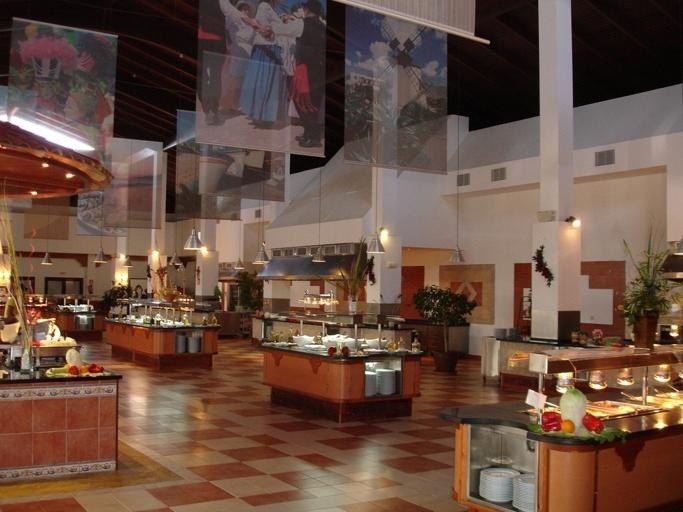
[253,152,272,264]
[40,199,54,266]
[168,139,208,267]
[233,219,246,271]
[554,364,673,394]
[565,215,582,228]
[91,187,136,268]
[366,165,387,254]
[311,159,328,264]
[450,115,468,264]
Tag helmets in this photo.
[175,334,202,354]
[305,345,326,352]
[262,342,296,348]
[265,313,299,322]
[361,361,401,397]
[394,352,409,356]
[478,467,536,512]
[362,349,388,355]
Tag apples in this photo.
[300,320,303,335]
[378,323,382,338]
[322,321,325,336]
[354,323,358,337]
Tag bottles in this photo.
[301,0,321,15]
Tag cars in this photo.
[163,324,167,328]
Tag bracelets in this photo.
[365,340,388,349]
[293,336,314,347]
[496,328,522,341]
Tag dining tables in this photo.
[412,285,479,373]
[616,216,683,354]
[315,230,373,316]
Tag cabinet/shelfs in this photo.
[211,316,217,325]
[411,335,420,352]
[202,316,208,327]
[384,336,406,353]
[183,314,189,328]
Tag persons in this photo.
[198,0,326,147]
[133,285,147,299]
[0,350,12,372]
[7,78,113,163]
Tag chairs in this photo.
[206,110,216,125]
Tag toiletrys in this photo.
[541,411,562,433]
[88,363,101,372]
[582,412,604,434]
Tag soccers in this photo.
[342,346,349,354]
[68,366,79,375]
[327,347,336,354]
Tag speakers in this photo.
[295,128,322,147]
